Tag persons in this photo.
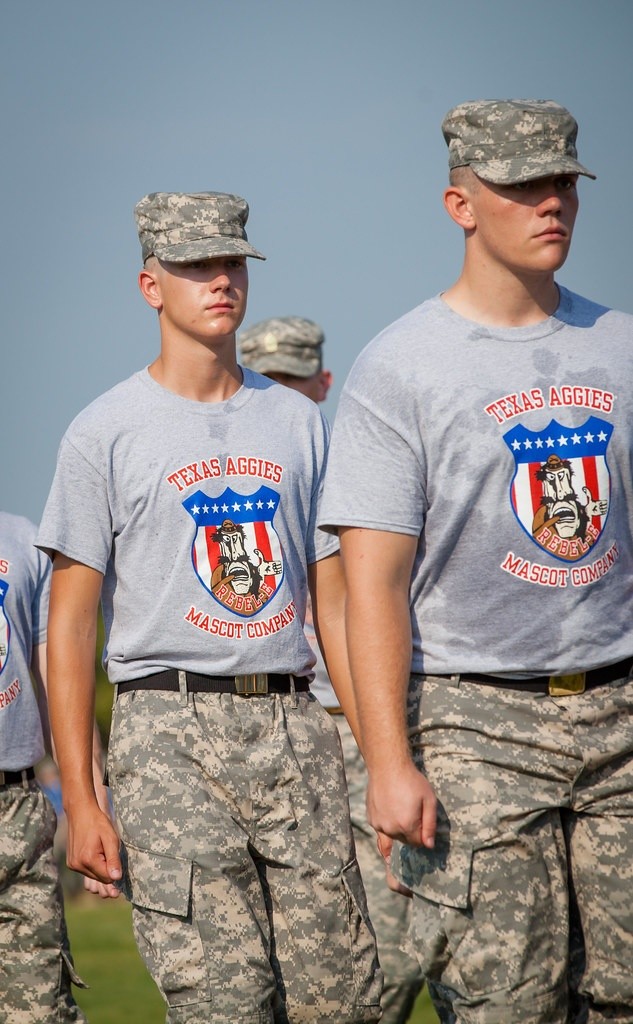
[0,511,122,1024]
[237,318,425,1024]
[36,193,381,1024]
[318,98,633,1024]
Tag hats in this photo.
[238,315,325,378]
[134,191,267,263]
[441,97,598,186]
